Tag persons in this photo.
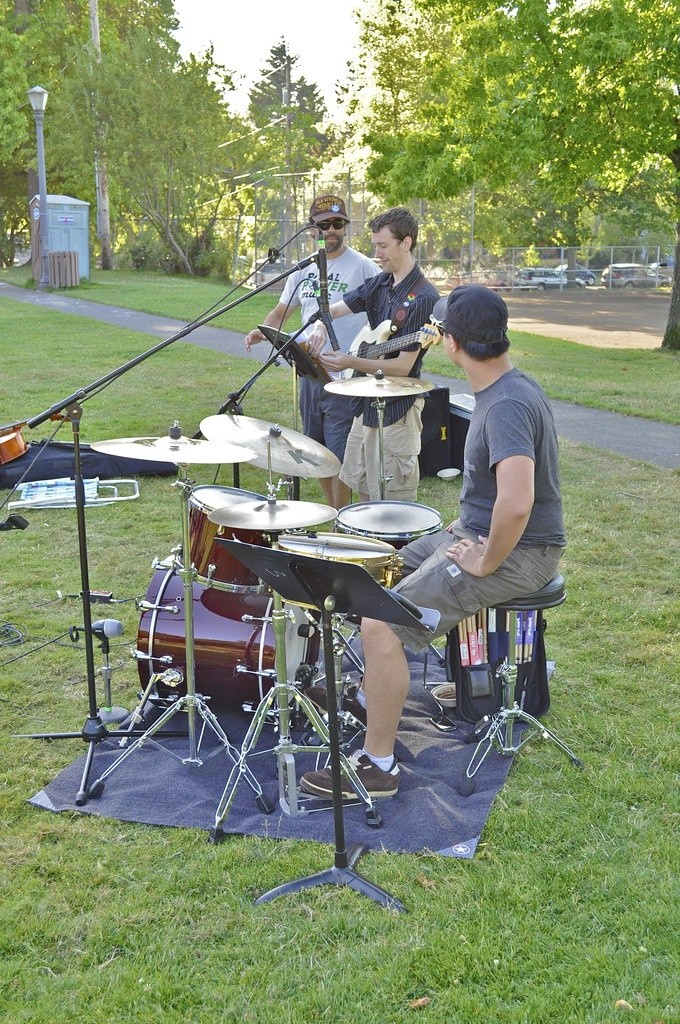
[306,205,441,506]
[295,282,564,801]
[245,195,382,535]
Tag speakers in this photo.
[417,384,451,479]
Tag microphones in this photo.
[317,231,329,314]
[79,619,123,638]
[312,280,341,352]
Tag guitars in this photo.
[343,314,443,381]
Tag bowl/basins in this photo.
[431,683,457,707]
[436,468,461,480]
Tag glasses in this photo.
[315,218,348,231]
[435,322,451,336]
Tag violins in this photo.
[0,413,73,467]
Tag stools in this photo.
[456,571,584,795]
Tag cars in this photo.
[514,267,534,284]
[600,263,672,289]
[554,263,596,286]
[648,262,667,270]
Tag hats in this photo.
[433,283,509,344]
[310,194,351,223]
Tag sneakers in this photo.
[299,748,401,800]
[303,682,367,730]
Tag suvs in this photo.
[519,267,586,291]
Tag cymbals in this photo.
[206,500,341,531]
[200,413,343,479]
[88,433,259,466]
[322,375,438,399]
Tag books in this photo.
[458,606,537,667]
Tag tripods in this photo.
[10,250,439,915]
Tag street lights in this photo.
[25,85,49,287]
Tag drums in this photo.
[138,555,323,719]
[173,483,270,595]
[334,498,443,552]
[274,529,397,618]
